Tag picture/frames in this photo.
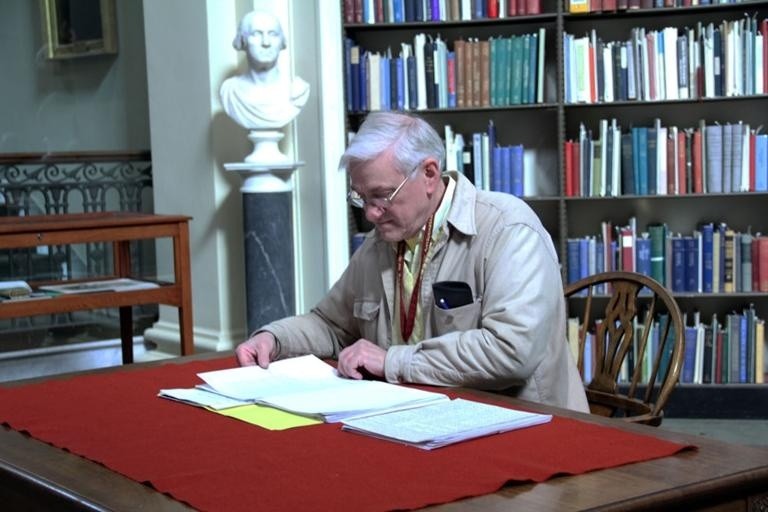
[39,0,118,64]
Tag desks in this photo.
[2,349,768,512]
[1,211,195,364]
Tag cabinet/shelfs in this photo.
[340,0,768,419]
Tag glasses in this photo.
[344,164,422,212]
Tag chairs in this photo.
[564,270,685,426]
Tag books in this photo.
[568,309,768,384]
[566,118,768,196]
[338,397,553,452]
[566,0,768,16]
[566,214,768,292]
[341,0,540,24]
[563,18,765,106]
[445,117,524,201]
[155,353,451,432]
[347,27,544,117]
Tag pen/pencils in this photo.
[440,299,448,309]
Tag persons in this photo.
[235,111,590,415]
[218,12,310,165]
